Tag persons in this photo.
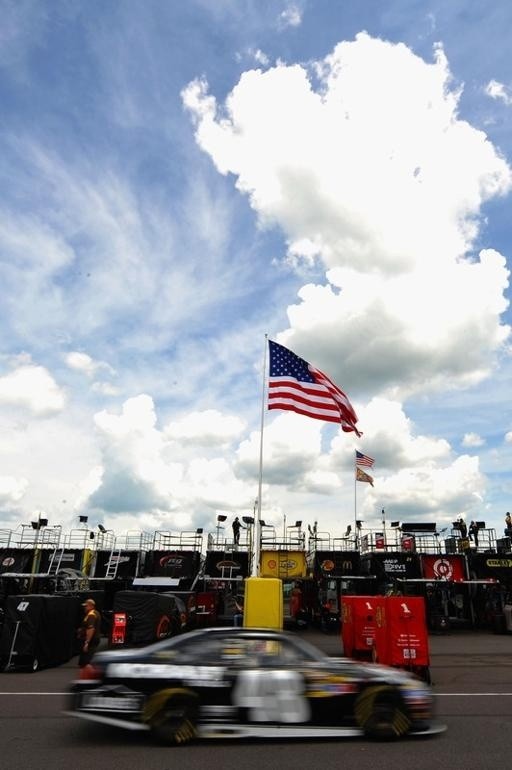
[289,584,303,617]
[233,517,244,544]
[505,512,512,528]
[468,521,478,546]
[77,599,101,669]
[460,519,467,538]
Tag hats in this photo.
[82,599,96,606]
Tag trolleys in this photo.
[4,621,40,673]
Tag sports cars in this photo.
[62,626,448,747]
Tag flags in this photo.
[356,467,374,487]
[268,340,363,438]
[355,450,375,468]
